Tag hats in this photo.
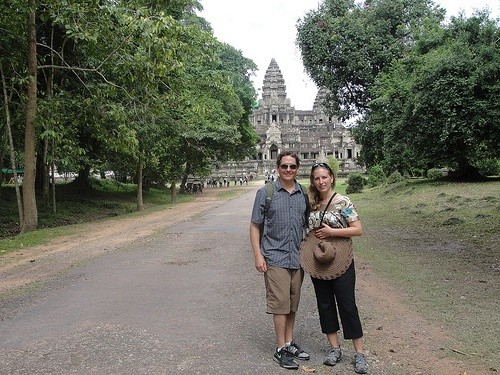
[299,227,354,279]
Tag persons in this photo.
[299,162,370,374]
[250,150,316,369]
[182,171,275,195]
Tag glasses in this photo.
[278,164,298,169]
[312,163,326,168]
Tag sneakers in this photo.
[355,352,369,374]
[323,345,343,366]
[285,340,310,360]
[273,345,299,369]
